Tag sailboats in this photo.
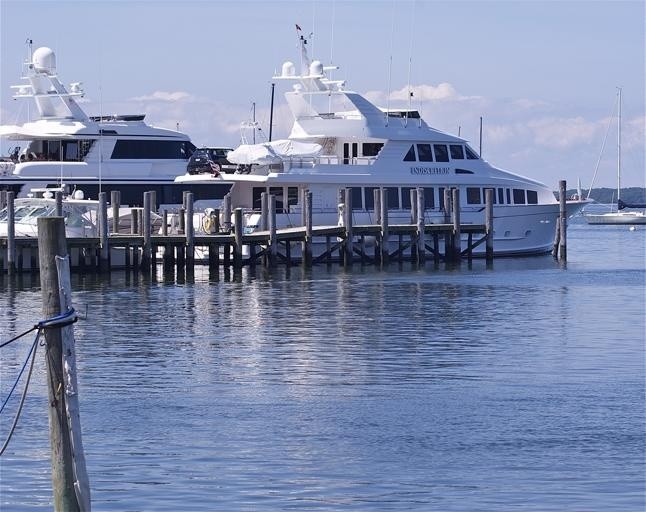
[580,87,646,224]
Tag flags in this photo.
[206,150,219,177]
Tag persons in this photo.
[9,153,45,164]
